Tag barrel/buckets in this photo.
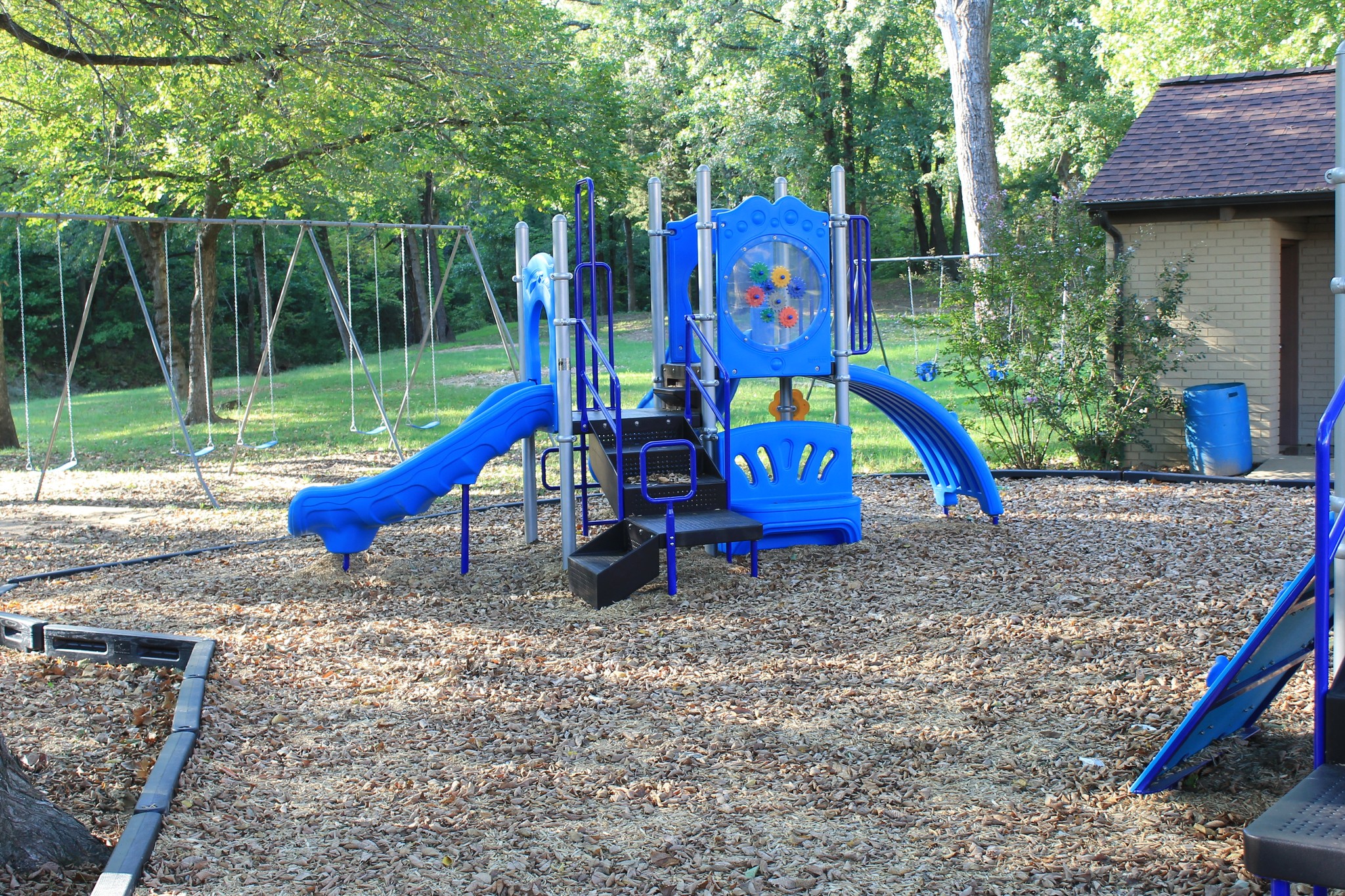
[1182,382,1253,476]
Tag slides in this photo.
[289,381,559,572]
[809,361,1004,524]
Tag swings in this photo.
[232,224,278,450]
[16,218,78,475]
[346,227,386,435]
[401,229,439,429]
[164,224,214,457]
